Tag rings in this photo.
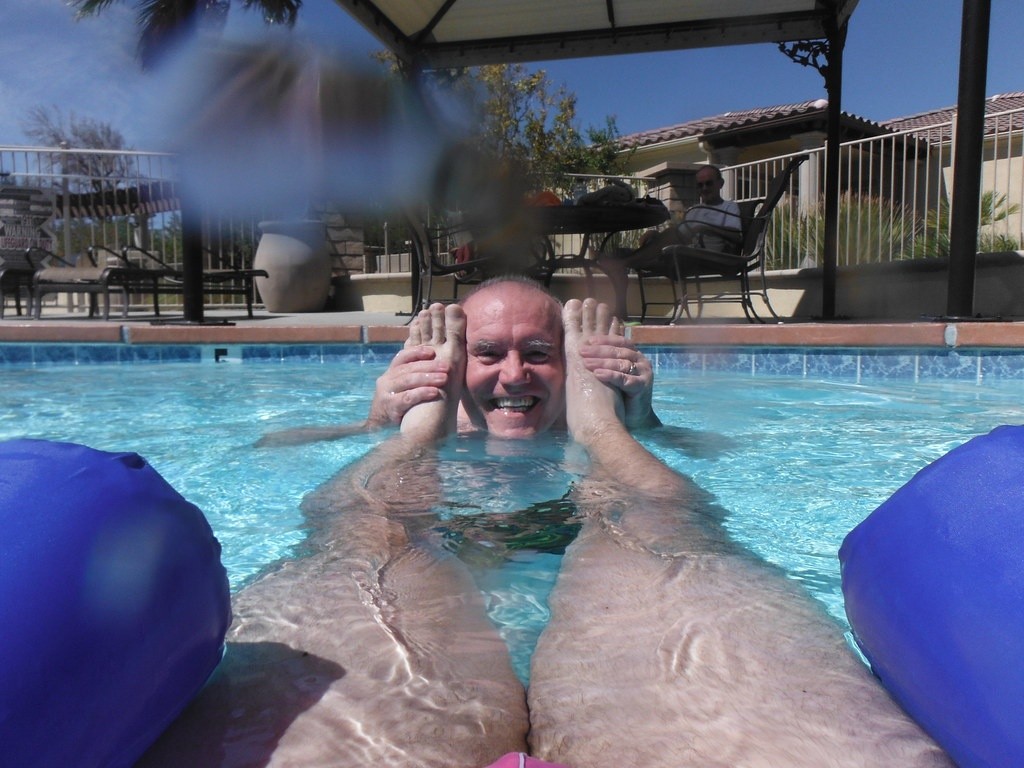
[627,362,636,374]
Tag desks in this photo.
[507,203,670,323]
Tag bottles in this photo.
[573,177,587,206]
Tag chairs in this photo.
[396,195,497,326]
[638,155,810,326]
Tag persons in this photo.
[598,165,742,321]
[369,276,686,493]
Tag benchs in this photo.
[0,246,162,319]
[33,243,269,319]
